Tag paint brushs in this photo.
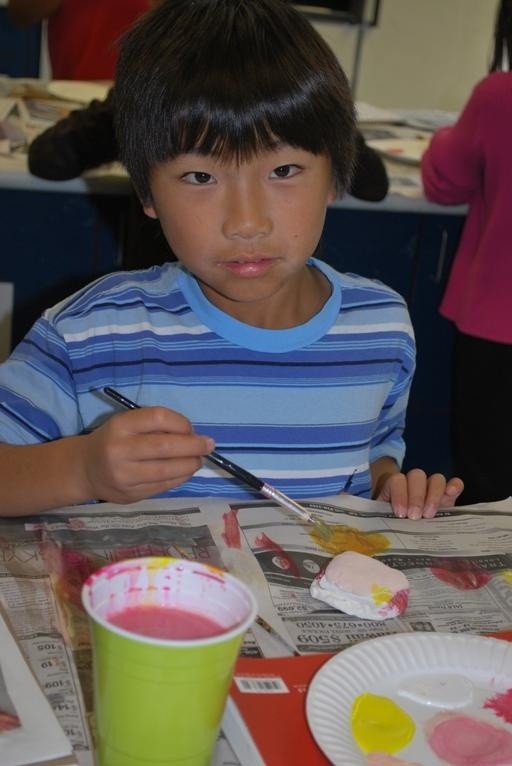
[104,387,333,541]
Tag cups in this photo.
[81,556,258,766]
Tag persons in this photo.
[2,0,154,81]
[418,2,512,504]
[31,82,390,203]
[1,2,465,522]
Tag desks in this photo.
[0,76,470,291]
[0,498,512,766]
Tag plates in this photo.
[366,137,428,163]
[46,79,107,105]
[305,632,512,766]
